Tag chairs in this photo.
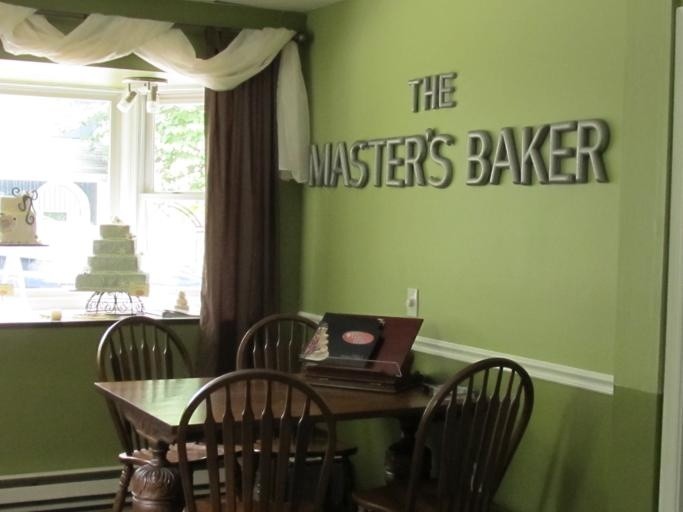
[350,355,539,511]
[93,310,247,512]
[173,368,339,512]
[230,309,366,512]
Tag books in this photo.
[419,379,476,402]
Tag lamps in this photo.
[116,76,168,115]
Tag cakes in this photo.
[1,196,37,245]
[74,219,147,291]
